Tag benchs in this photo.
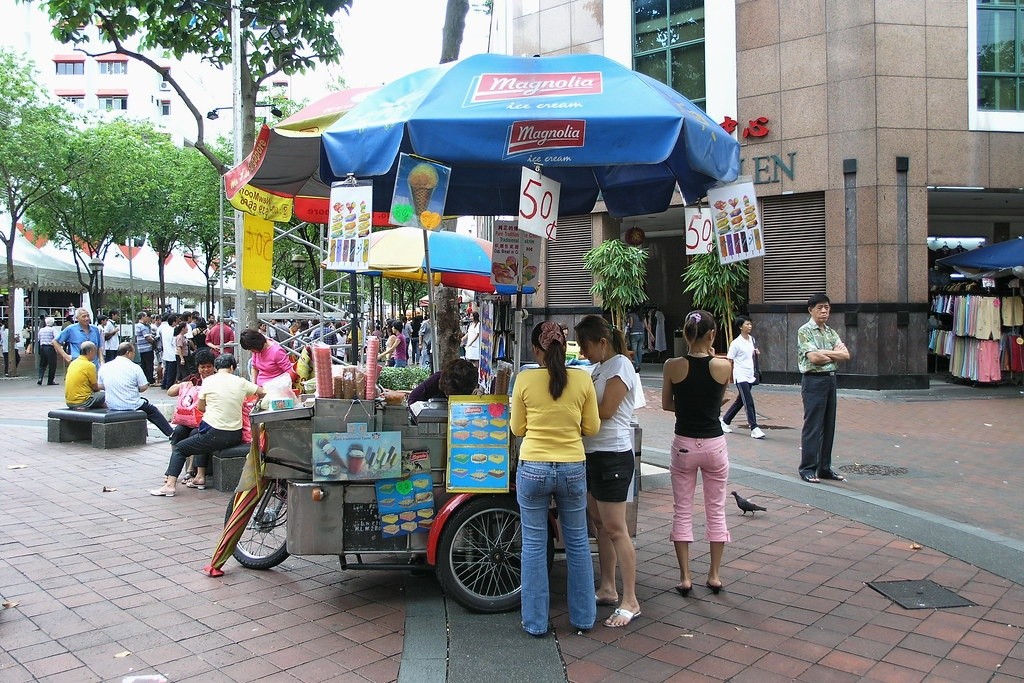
[47,408,148,450]
[211,441,250,491]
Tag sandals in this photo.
[803,474,821,483]
[818,471,846,481]
[181,470,196,484]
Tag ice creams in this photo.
[322,444,349,469]
[506,255,537,285]
[408,163,438,216]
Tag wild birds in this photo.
[731,490,767,516]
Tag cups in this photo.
[348,456,364,475]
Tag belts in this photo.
[806,370,838,376]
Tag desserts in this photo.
[452,419,509,480]
[378,479,435,534]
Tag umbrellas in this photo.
[320,225,495,371]
[221,53,740,368]
[934,232,1024,334]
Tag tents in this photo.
[419,287,462,319]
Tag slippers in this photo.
[593,593,623,606]
[150,489,176,497]
[186,481,208,490]
[601,609,642,628]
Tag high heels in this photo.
[705,578,723,595]
[675,583,693,598]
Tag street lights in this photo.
[208,274,218,315]
[88,257,105,325]
[291,253,307,312]
[373,281,380,319]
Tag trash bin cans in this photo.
[674,330,687,356]
[513,436,643,553]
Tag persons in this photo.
[718,316,767,438]
[407,358,478,407]
[509,320,602,636]
[150,353,266,498]
[627,302,656,372]
[0,293,77,387]
[51,304,234,391]
[239,330,295,393]
[575,315,646,628]
[661,309,734,600]
[164,349,219,484]
[796,292,851,483]
[64,339,107,409]
[96,342,175,442]
[460,311,481,361]
[260,310,434,374]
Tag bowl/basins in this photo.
[383,392,406,406]
[314,348,333,397]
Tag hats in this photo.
[45,316,54,326]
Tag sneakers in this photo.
[750,427,766,439]
[719,417,734,433]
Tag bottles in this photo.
[235,364,241,376]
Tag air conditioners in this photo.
[160,81,170,91]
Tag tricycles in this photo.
[222,394,644,615]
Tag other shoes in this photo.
[150,382,162,387]
[47,381,60,386]
[37,379,44,385]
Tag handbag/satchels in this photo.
[169,375,204,428]
[752,368,764,385]
[240,393,259,444]
